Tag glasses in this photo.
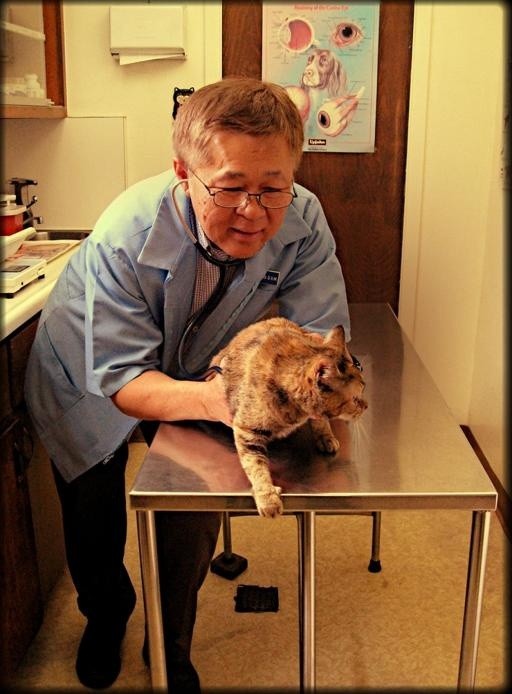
[186,166,298,209]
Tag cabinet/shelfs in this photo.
[0,0,68,118]
[0,315,66,690]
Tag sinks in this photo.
[26,227,94,242]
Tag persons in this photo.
[23,78,351,693]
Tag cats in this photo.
[203,317,375,520]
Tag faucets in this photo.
[22,212,44,227]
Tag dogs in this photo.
[300,48,347,100]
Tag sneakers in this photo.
[141,640,200,694]
[75,582,137,690]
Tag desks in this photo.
[130,302,499,694]
